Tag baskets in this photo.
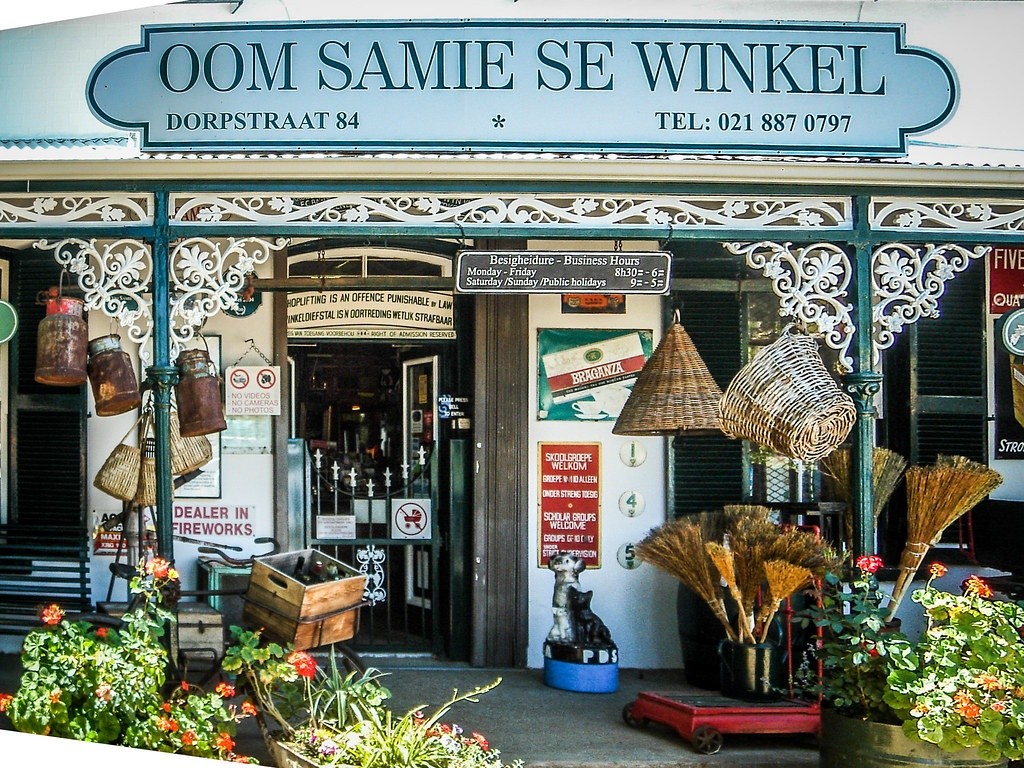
[715,323,858,462]
[93,410,147,501]
[170,397,212,475]
[132,413,175,507]
[612,309,726,437]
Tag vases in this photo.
[274,739,320,768]
[821,696,1007,768]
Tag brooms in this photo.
[628,441,1004,704]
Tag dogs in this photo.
[545,551,586,642]
[568,585,617,651]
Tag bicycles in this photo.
[58,536,382,734]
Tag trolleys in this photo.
[622,522,840,755]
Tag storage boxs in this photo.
[93,408,213,508]
[715,321,858,467]
[241,546,368,651]
[607,308,736,440]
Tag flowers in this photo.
[296,676,524,768]
[803,557,1023,760]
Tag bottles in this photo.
[85,333,140,420]
[175,348,229,440]
[35,294,89,387]
